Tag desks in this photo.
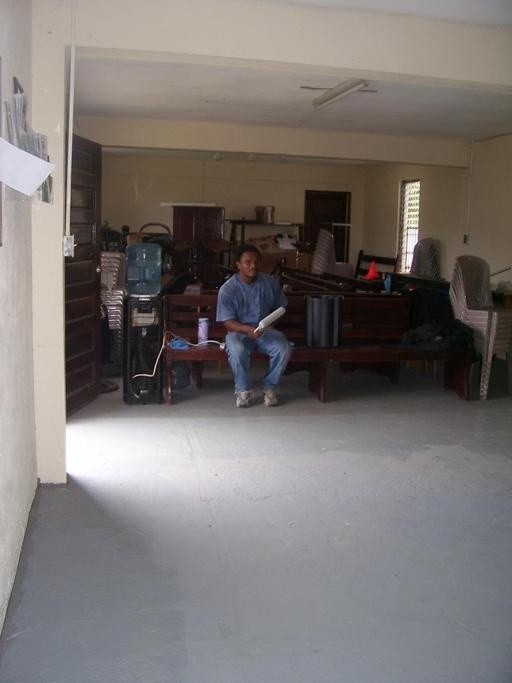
[391,272,505,392]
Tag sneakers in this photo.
[262,388,280,407]
[235,390,253,408]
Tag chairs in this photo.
[100,250,125,366]
[311,228,336,273]
[449,255,512,400]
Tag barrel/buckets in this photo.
[304,294,345,347]
[255,205,275,224]
[124,241,163,296]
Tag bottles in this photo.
[197,318,209,346]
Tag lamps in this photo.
[311,77,369,112]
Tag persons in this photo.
[122,225,129,232]
[216,246,294,408]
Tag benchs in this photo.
[277,265,402,296]
[162,293,480,404]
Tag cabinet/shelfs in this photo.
[228,216,305,269]
[318,220,351,263]
